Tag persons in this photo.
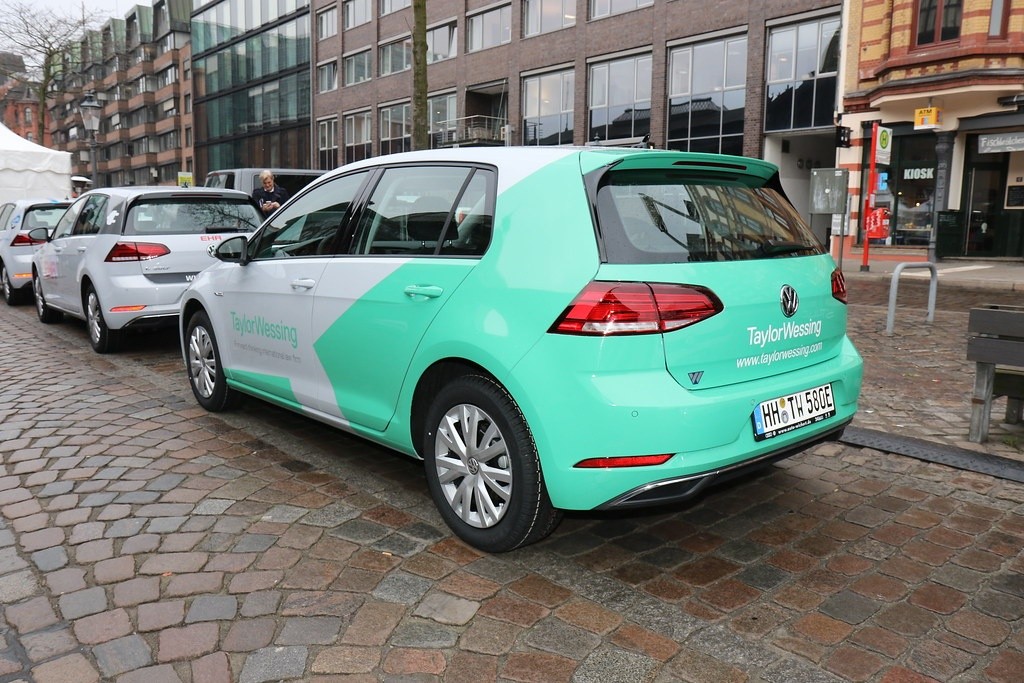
[251,168,291,219]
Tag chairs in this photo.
[402,195,460,241]
[174,206,206,230]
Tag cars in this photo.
[0,197,78,307]
[27,185,266,355]
[206,167,330,206]
[183,146,863,553]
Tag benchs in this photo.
[964,303,1024,444]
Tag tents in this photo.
[0,120,73,205]
